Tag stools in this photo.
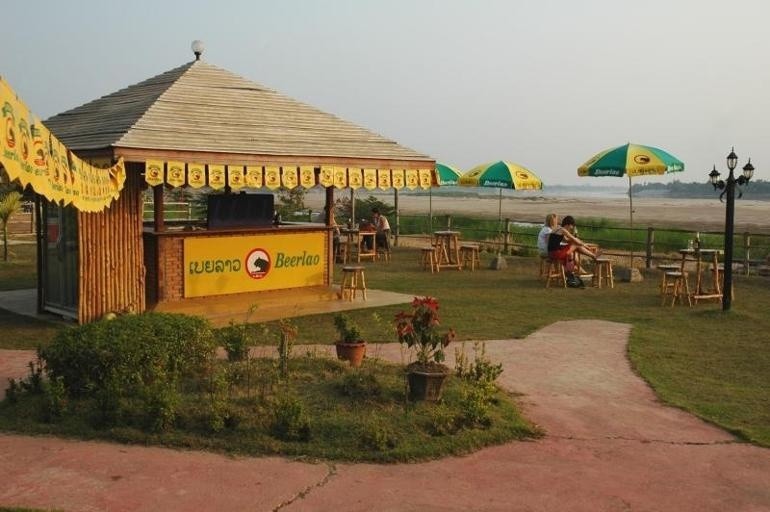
[420,242,481,273]
[334,242,352,265]
[341,266,367,301]
[539,255,614,289]
[657,265,734,307]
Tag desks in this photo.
[559,242,599,286]
[430,231,461,271]
[339,231,377,262]
[679,247,734,305]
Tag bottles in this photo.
[347,217,353,229]
[360,219,365,229]
[694,232,701,251]
[572,225,579,238]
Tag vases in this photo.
[407,362,451,403]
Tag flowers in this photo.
[393,295,457,366]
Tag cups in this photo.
[687,240,694,250]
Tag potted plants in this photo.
[214,303,258,362]
[333,312,367,369]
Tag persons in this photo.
[317,205,345,263]
[549,215,604,277]
[536,213,588,274]
[363,207,392,251]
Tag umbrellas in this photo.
[578,141,686,283]
[424,163,463,247]
[461,159,545,270]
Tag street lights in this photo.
[710,144,755,314]
